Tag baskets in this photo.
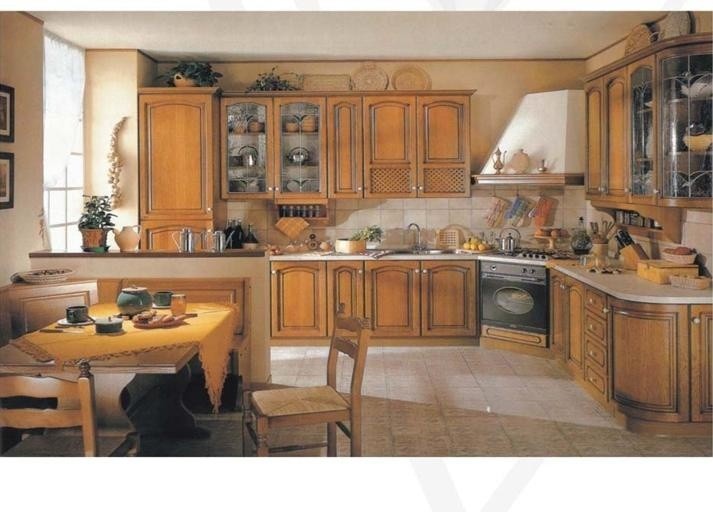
[661,249,698,264]
[670,275,710,289]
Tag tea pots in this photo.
[285,147,312,166]
[235,146,258,166]
[494,227,521,254]
[117,284,153,316]
[170,227,203,255]
[490,146,507,176]
[111,224,142,252]
[535,158,549,174]
[208,230,235,256]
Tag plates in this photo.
[57,317,95,326]
[151,303,173,309]
[133,316,184,328]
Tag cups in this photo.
[170,294,186,317]
[65,305,88,323]
[578,254,594,268]
[153,291,172,306]
[594,256,610,268]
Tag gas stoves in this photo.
[495,247,551,260]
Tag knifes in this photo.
[613,231,634,248]
[39,328,85,335]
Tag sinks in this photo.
[418,246,459,257]
[372,247,418,258]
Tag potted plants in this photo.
[151,56,224,86]
[78,193,119,253]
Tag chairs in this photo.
[0,359,141,457]
[241,303,373,456]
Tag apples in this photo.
[463,237,493,251]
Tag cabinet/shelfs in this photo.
[550,269,712,434]
[626,32,713,210]
[218,91,326,201]
[582,55,635,205]
[137,86,227,252]
[327,89,364,198]
[269,261,479,346]
[363,89,477,198]
[1,277,253,391]
[273,199,337,228]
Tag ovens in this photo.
[478,260,549,348]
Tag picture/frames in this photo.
[0,82,15,142]
[0,151,15,209]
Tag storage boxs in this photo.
[299,74,352,92]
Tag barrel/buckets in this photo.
[407,222,421,249]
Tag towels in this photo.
[274,216,310,241]
